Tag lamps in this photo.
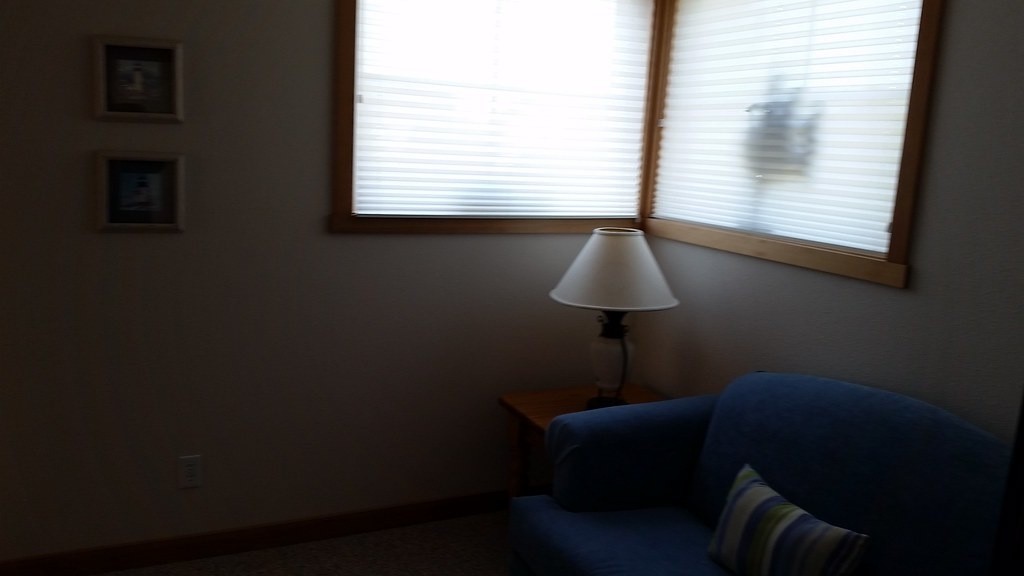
[548,226,679,412]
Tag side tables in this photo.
[500,387,670,498]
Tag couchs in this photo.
[503,371,1024,576]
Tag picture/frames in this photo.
[94,34,184,123]
[94,149,186,234]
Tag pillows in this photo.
[706,462,871,576]
